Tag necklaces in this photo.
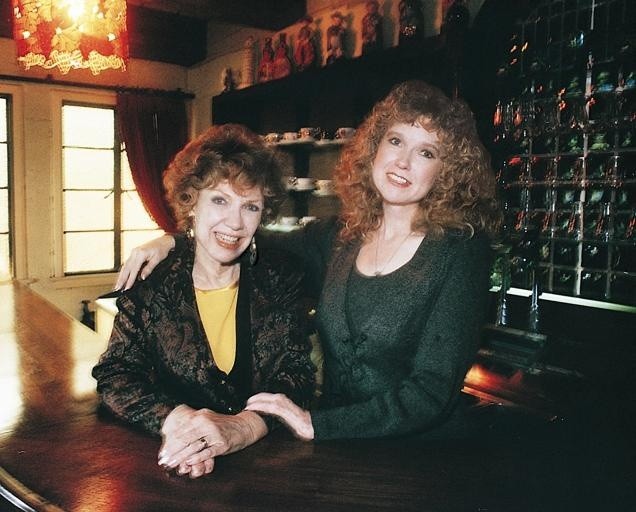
[373,226,416,279]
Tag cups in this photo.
[278,131,298,140]
[337,127,357,139]
[281,216,299,226]
[298,215,318,227]
[300,127,319,140]
[318,179,337,194]
[297,177,319,188]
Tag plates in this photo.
[295,186,316,192]
[314,189,336,198]
[277,225,301,233]
[297,136,318,143]
[278,138,298,145]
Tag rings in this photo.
[199,438,208,449]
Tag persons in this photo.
[108,79,507,447]
[257,0,425,84]
[87,121,317,481]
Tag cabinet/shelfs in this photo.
[210,33,435,199]
